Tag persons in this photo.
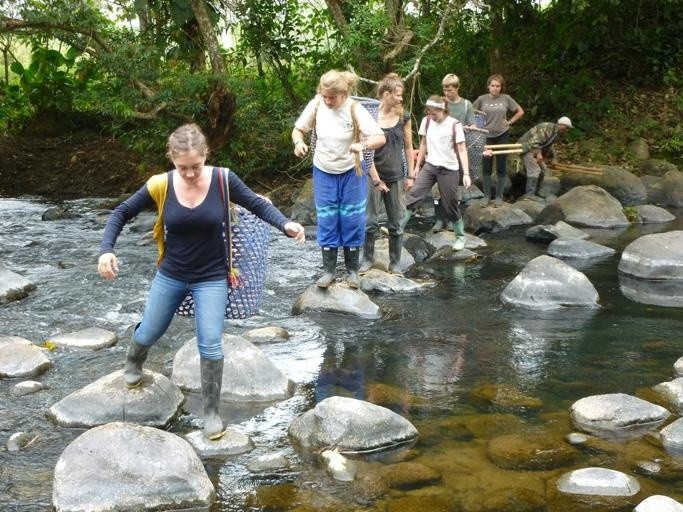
[359,72,414,280]
[381,95,471,251]
[471,74,524,208]
[291,69,387,290]
[515,116,574,201]
[441,72,477,134]
[96,123,306,441]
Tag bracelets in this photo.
[462,173,469,177]
[508,120,512,125]
[373,180,381,186]
[358,140,368,152]
[293,138,302,145]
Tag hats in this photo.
[558,117,575,129]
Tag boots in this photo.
[316,175,546,289]
[200,357,226,440]
[123,322,149,388]
